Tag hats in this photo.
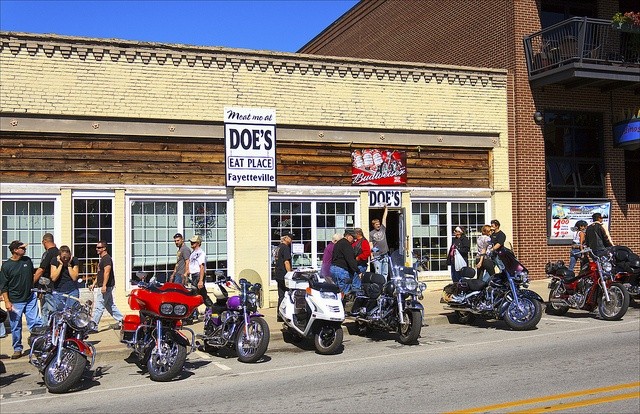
[282,230,295,238]
[344,228,357,238]
[188,235,202,244]
[9,240,28,251]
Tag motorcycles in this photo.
[545,240,630,320]
[341,246,429,345]
[439,241,545,331]
[27,276,96,394]
[194,270,270,363]
[119,271,208,382]
[277,264,346,354]
[604,245,640,309]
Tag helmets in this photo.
[214,284,228,301]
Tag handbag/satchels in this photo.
[353,237,366,256]
[571,230,586,257]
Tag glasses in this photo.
[95,247,105,249]
[173,235,183,239]
[454,229,459,232]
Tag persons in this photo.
[352,226,373,274]
[275,229,296,321]
[328,226,366,307]
[168,232,199,324]
[181,234,214,310]
[568,219,589,273]
[585,212,616,262]
[487,218,507,272]
[0,239,44,359]
[87,238,125,333]
[475,224,496,279]
[50,245,80,324]
[446,225,470,282]
[368,203,391,286]
[321,231,344,284]
[32,232,62,339]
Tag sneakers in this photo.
[11,350,22,359]
